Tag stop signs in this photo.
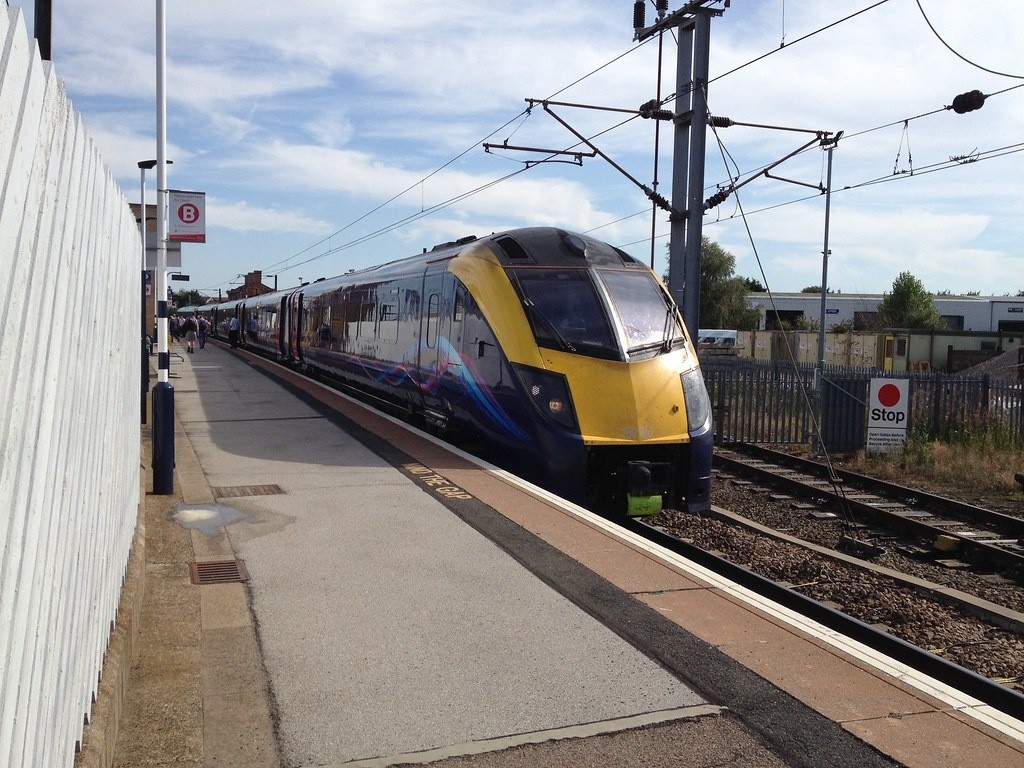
[870,378,907,428]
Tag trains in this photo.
[175,227,713,515]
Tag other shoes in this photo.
[234,346,237,349]
[229,345,234,348]
[178,338,180,342]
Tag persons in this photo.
[249,316,259,338]
[230,315,239,348]
[169,313,185,342]
[198,316,211,349]
[186,316,199,353]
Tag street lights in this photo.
[137,158,174,426]
[810,130,844,454]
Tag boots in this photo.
[187,346,194,353]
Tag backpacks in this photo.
[200,319,207,330]
